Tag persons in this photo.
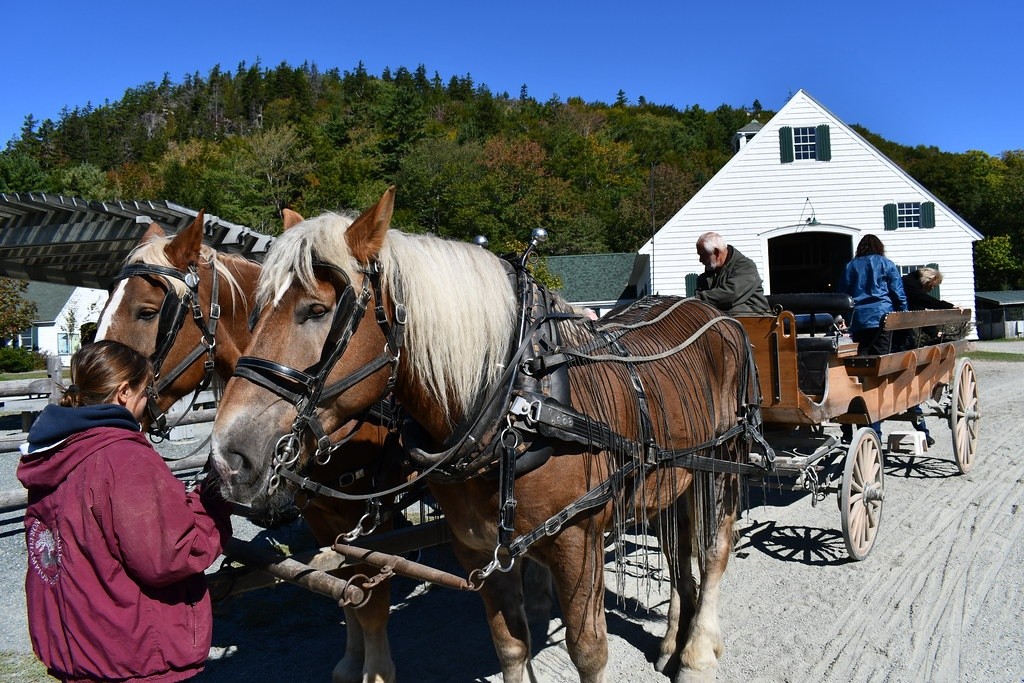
[844,234,907,358]
[899,269,963,349]
[694,233,771,315]
[15,340,222,683]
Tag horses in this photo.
[88,209,535,683]
[209,185,753,683]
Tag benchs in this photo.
[765,294,854,396]
[842,310,974,391]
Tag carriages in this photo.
[90,183,987,683]
